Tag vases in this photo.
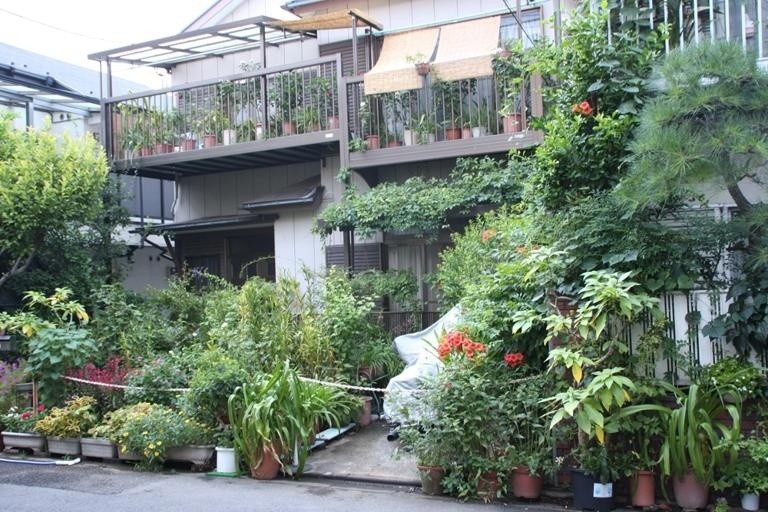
[256,122,264,140]
[3,433,44,454]
[113,436,215,467]
[470,457,544,502]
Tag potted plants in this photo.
[180,120,196,150]
[276,74,302,134]
[422,118,435,143]
[118,106,153,159]
[338,291,374,427]
[216,111,237,145]
[293,381,331,446]
[547,277,765,510]
[459,114,471,139]
[403,106,421,146]
[393,403,456,497]
[195,113,219,147]
[81,420,115,460]
[37,396,96,458]
[321,81,341,129]
[151,111,172,153]
[365,111,380,149]
[385,131,402,147]
[0,312,8,335]
[446,113,460,140]
[189,348,245,475]
[303,108,319,132]
[501,98,524,133]
[227,367,314,479]
[471,101,490,137]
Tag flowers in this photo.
[4,404,47,435]
[430,330,548,468]
[112,404,203,460]
